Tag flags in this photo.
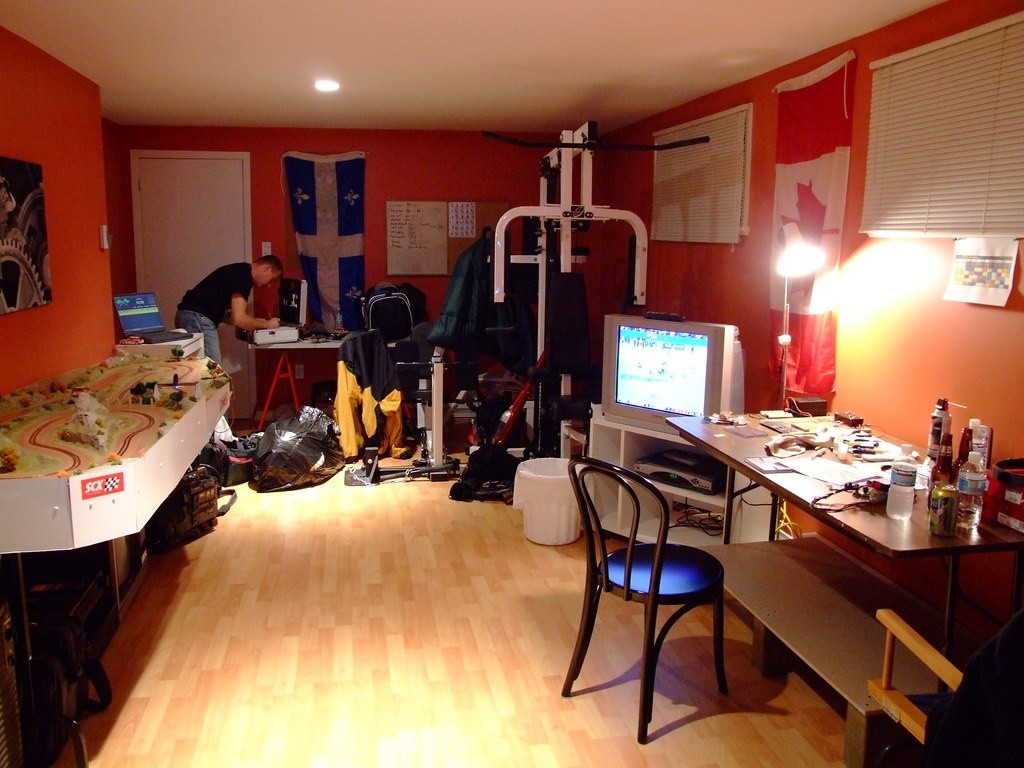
[281,150,366,332]
[766,49,856,396]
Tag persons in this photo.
[174,254,283,437]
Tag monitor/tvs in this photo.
[601,313,745,436]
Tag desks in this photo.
[249,332,413,429]
[116,333,206,360]
[0,358,235,695]
[665,412,1024,767]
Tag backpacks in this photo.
[361,283,413,342]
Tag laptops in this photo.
[113,291,194,345]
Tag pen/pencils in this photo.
[268,313,272,319]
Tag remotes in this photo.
[664,450,701,466]
[645,311,686,322]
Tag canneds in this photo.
[927,483,960,538]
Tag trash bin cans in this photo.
[511,458,595,545]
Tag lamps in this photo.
[775,222,830,421]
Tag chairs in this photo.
[869,607,1024,768]
[561,456,729,743]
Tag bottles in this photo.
[886,445,918,520]
[969,419,985,459]
[335,307,342,328]
[956,453,985,529]
[954,428,973,486]
[360,296,366,317]
[925,398,953,462]
[926,433,957,510]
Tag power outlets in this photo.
[293,364,305,379]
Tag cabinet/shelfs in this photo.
[591,416,772,548]
